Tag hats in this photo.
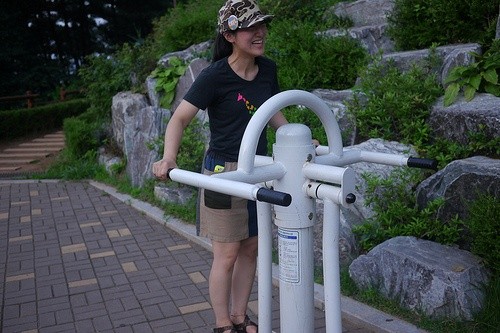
[218,0,274,34]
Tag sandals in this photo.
[213,316,257,333]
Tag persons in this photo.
[152,0,322,333]
